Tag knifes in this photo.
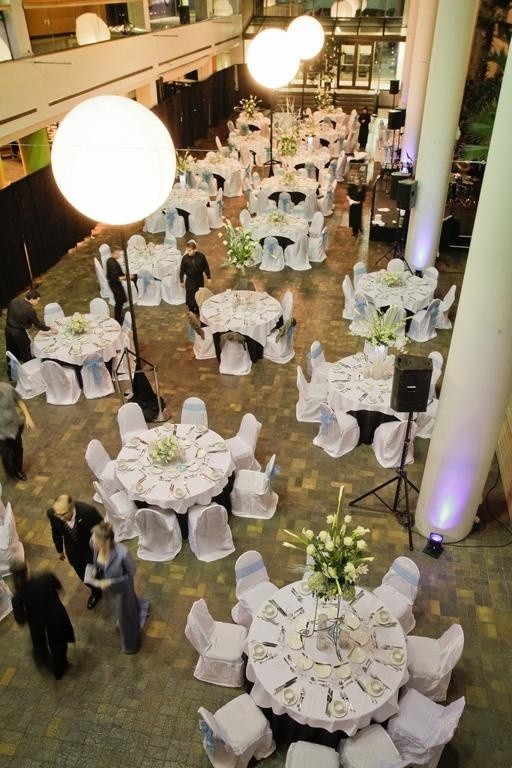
[273,676,297,696]
[325,687,334,717]
[355,680,377,704]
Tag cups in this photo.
[118,422,229,499]
[333,700,344,713]
[283,688,294,704]
[250,582,406,688]
[369,679,382,692]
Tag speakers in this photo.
[388,109,399,130]
[395,107,405,127]
[389,79,399,94]
[390,354,433,413]
[390,171,412,200]
[397,178,418,209]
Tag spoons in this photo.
[371,673,390,690]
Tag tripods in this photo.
[374,217,411,268]
[373,129,401,193]
[346,422,422,553]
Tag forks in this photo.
[296,687,305,712]
[339,691,356,712]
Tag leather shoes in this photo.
[86,591,103,610]
[16,469,27,481]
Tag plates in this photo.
[357,269,429,302]
[37,314,121,354]
[366,681,384,697]
[332,350,389,406]
[329,701,347,718]
[200,289,281,327]
[119,242,181,272]
[281,692,297,705]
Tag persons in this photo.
[85,521,151,654]
[180,242,211,315]
[345,176,368,235]
[47,495,105,610]
[358,108,371,150]
[5,290,58,381]
[106,247,134,324]
[1,382,36,481]
[7,556,76,680]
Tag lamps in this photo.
[286,14,325,119]
[245,26,299,177]
[52,94,174,228]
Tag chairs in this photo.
[80,396,284,568]
[93,234,196,308]
[296,340,446,469]
[142,91,362,234]
[3,297,139,405]
[343,259,457,345]
[188,284,295,374]
[223,189,328,274]
[182,547,471,768]
